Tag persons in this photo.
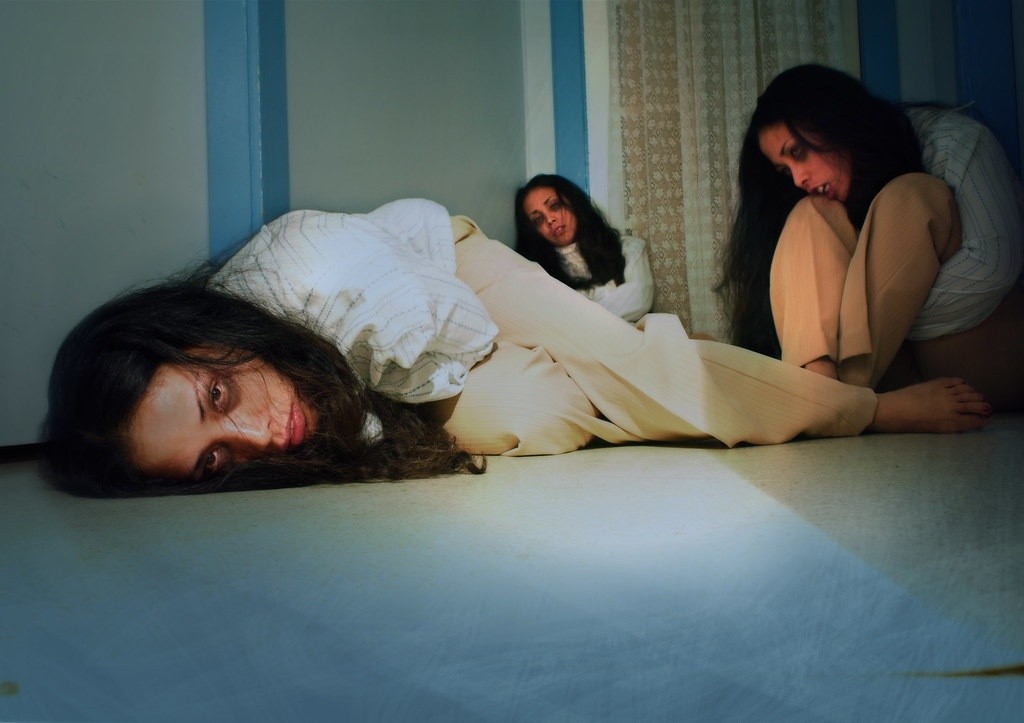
[712,64,1024,411]
[514,174,717,343]
[42,199,992,500]
